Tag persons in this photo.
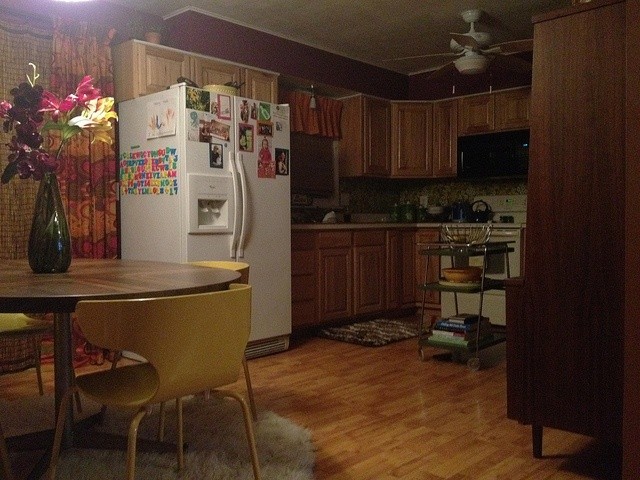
[277,151,287,173]
[211,145,220,163]
[245,128,253,150]
[259,136,272,167]
[240,127,246,150]
[260,124,270,133]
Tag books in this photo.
[428,313,492,347]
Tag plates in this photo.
[439,281,484,288]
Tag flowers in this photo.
[1,63,121,220]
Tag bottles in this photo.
[344,192,351,222]
[389,202,400,222]
[401,199,415,221]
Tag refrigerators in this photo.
[117,82,292,361]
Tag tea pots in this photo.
[470,200,494,222]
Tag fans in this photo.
[397,9,533,75]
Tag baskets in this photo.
[1,324,46,371]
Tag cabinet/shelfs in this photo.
[364,97,391,176]
[458,87,530,135]
[386,230,415,311]
[115,37,281,105]
[505,0,640,459]
[434,99,458,175]
[414,240,517,372]
[417,228,440,305]
[290,233,316,326]
[390,103,434,177]
[318,231,385,326]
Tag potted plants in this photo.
[440,224,493,283]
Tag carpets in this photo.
[0,367,325,480]
[320,318,430,346]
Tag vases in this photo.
[28,172,71,273]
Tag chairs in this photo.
[0,312,56,397]
[46,282,263,479]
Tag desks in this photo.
[0,257,242,418]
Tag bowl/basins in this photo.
[203,85,238,97]
[442,266,483,283]
